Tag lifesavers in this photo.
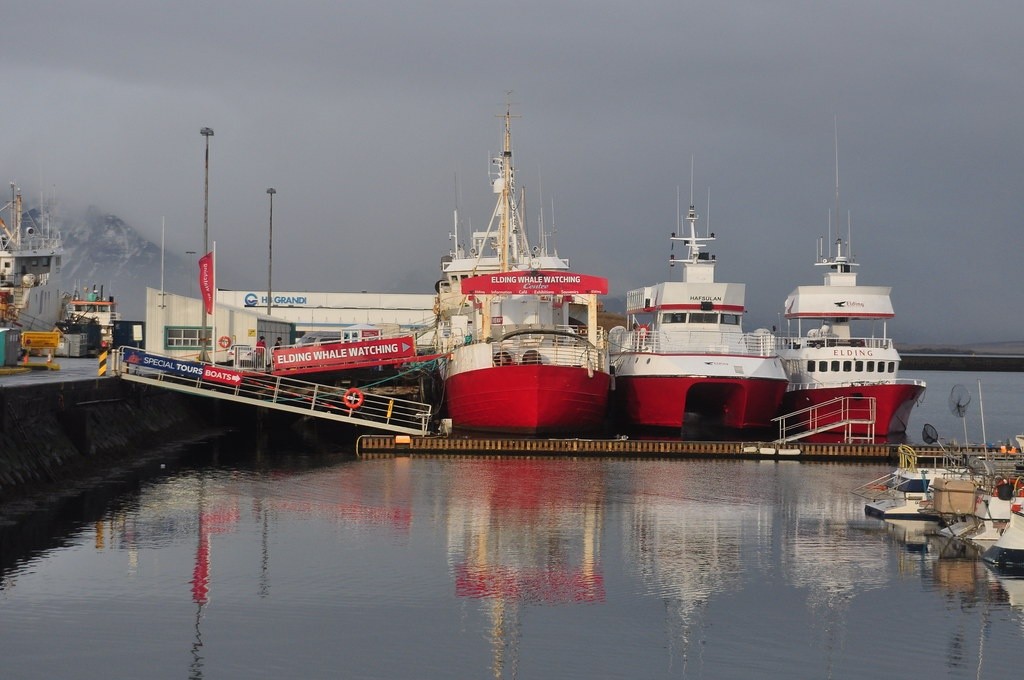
[522,349,542,365]
[343,388,364,409]
[494,351,512,367]
[218,335,232,349]
[993,478,1024,512]
[638,324,650,341]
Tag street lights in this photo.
[266,186,276,314]
[200,126,216,364]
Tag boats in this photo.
[852,444,1024,579]
[269,86,617,437]
[609,153,786,430]
[754,111,926,448]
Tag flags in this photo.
[197,251,214,314]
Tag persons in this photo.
[83,287,100,310]
[256,336,265,368]
[274,337,282,351]
